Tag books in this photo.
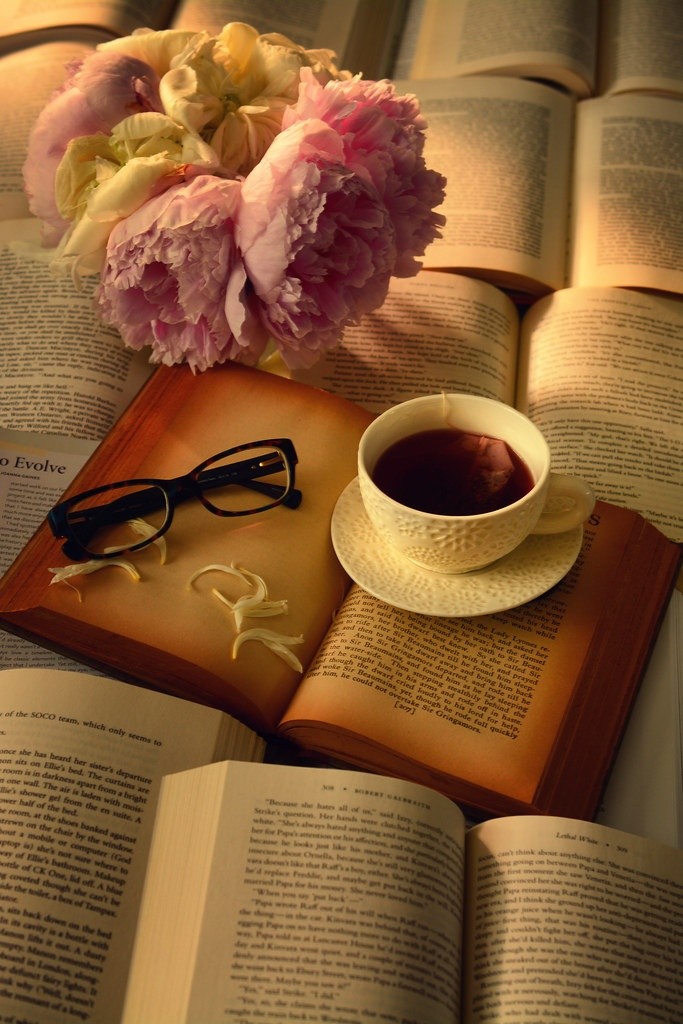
[0,0,683,1024]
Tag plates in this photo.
[331,473,584,616]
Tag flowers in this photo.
[20,20,453,373]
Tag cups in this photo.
[358,394,550,575]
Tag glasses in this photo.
[46,438,302,562]
